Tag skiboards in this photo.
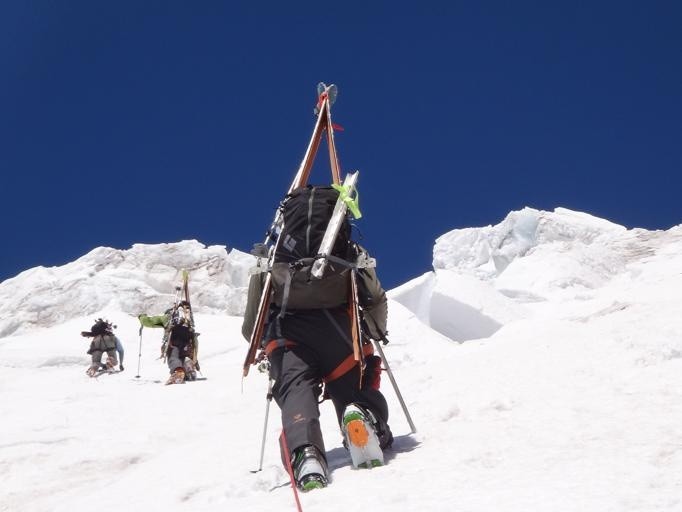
[242,81,366,388]
[158,269,200,372]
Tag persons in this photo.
[242,246,388,493]
[138,301,193,385]
[87,333,124,376]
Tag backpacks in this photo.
[270,183,353,310]
[91,318,116,335]
[171,301,192,350]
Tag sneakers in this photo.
[342,403,386,469]
[293,445,328,488]
